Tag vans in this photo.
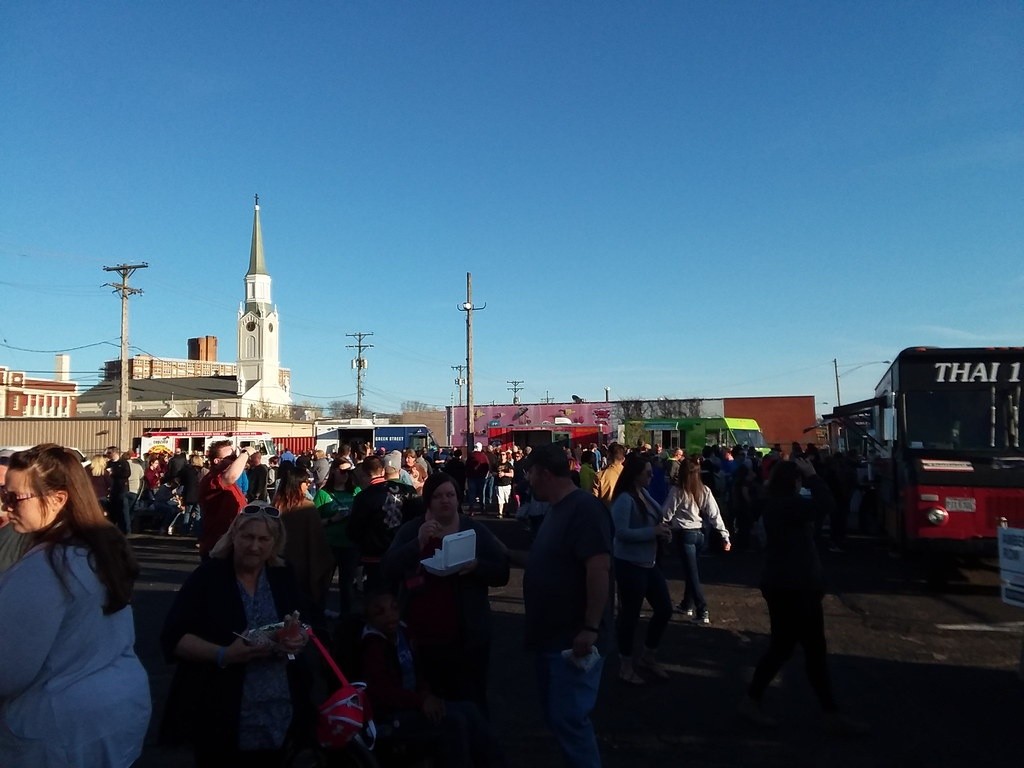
[0,445,91,470]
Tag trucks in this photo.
[822,345,1023,569]
[624,416,773,459]
[337,423,440,462]
[140,432,281,469]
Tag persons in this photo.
[497,436,619,768]
[382,475,510,729]
[357,586,510,768]
[88,442,893,741]
[0,442,152,768]
[157,499,311,768]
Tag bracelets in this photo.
[582,627,601,634]
[504,473,506,476]
[215,646,230,669]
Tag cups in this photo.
[243,629,267,649]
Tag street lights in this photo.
[835,357,893,406]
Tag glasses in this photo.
[0,491,49,509]
[107,452,114,454]
[234,504,281,536]
[219,454,237,461]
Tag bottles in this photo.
[281,611,303,649]
[273,615,291,642]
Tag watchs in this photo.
[327,517,333,526]
[241,450,250,456]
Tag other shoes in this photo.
[168,526,174,535]
[496,513,503,519]
[469,512,474,517]
[820,699,841,715]
[751,708,778,729]
[690,617,712,626]
[639,653,672,680]
[617,664,646,684]
[675,605,692,616]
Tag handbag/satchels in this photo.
[313,680,377,751]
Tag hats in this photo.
[422,472,460,509]
[381,453,401,474]
[474,442,482,452]
